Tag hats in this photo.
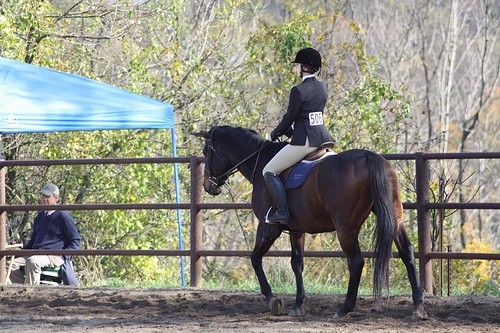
[38,183,59,197]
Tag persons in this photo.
[259,47,336,222]
[6,183,81,286]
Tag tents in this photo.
[0,56,184,286]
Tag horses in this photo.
[187,123,430,322]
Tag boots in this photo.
[263,171,291,224]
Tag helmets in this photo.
[291,47,321,66]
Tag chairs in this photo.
[20,266,63,286]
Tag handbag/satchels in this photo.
[60,256,77,286]
